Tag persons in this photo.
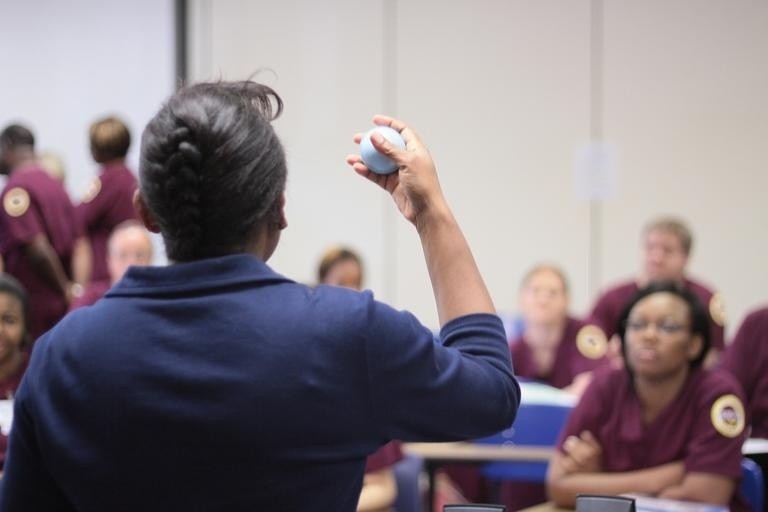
[357,439,404,512]
[2,80,520,512]
[547,285,753,512]
[1,118,151,463]
[590,215,726,368]
[317,248,363,291]
[504,266,607,508]
[717,304,768,442]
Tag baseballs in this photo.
[359,126,406,174]
